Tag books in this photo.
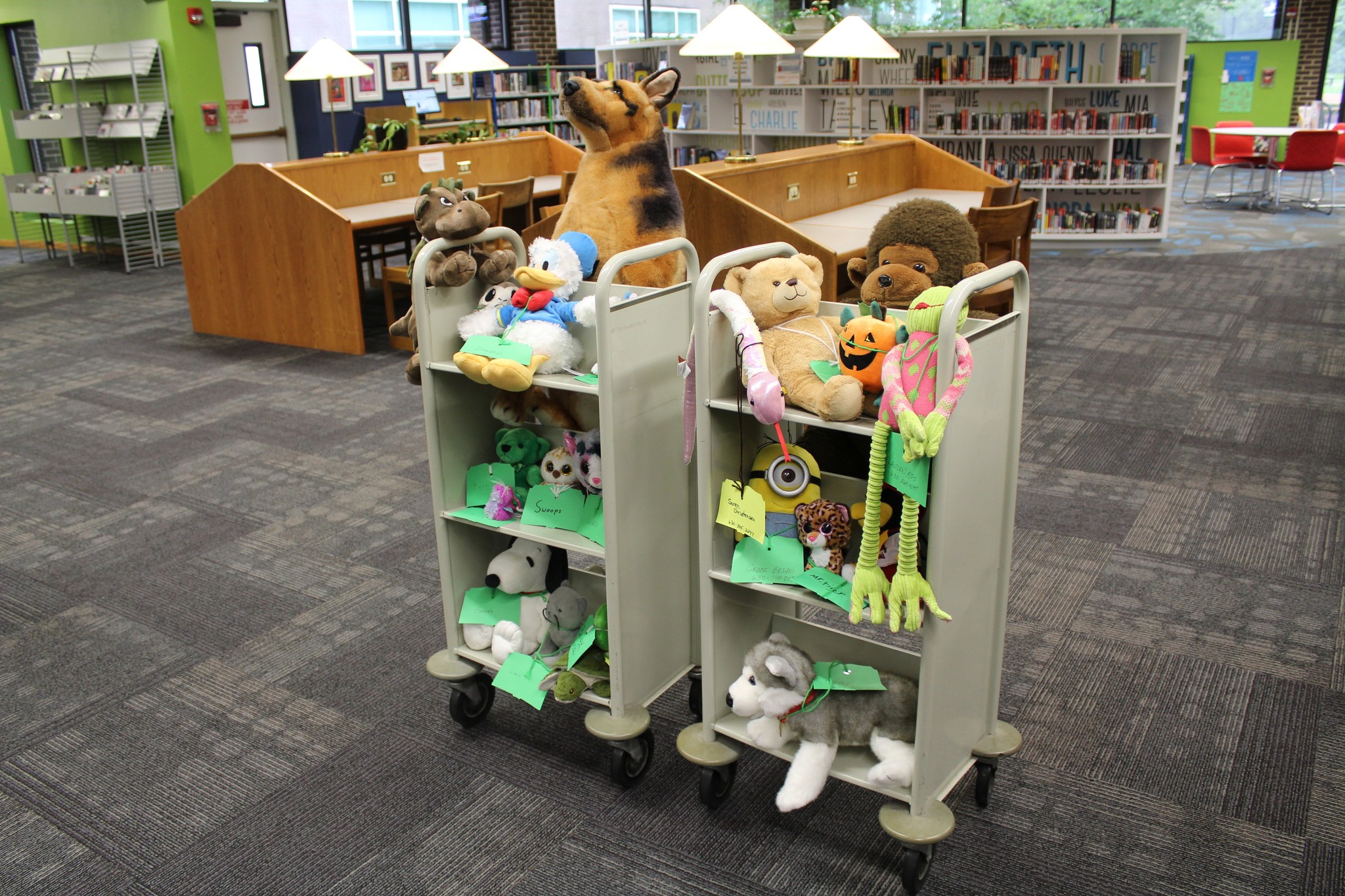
[887,51,1167,233]
[491,58,753,169]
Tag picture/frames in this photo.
[419,53,447,94]
[446,72,473,99]
[353,54,383,102]
[319,78,353,113]
[383,53,418,91]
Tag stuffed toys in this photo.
[841,478,928,611]
[844,197,1003,324]
[837,302,911,395]
[547,66,690,288]
[678,287,794,468]
[733,420,823,544]
[849,284,974,634]
[722,252,882,422]
[384,177,518,388]
[726,631,921,814]
[793,499,850,574]
[454,231,640,703]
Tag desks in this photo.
[521,134,1011,303]
[1207,127,1329,211]
[364,99,496,148]
[173,132,588,356]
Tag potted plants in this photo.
[774,1,844,34]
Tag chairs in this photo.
[1182,125,1255,211]
[1213,121,1275,203]
[1300,122,1345,208]
[968,177,1040,313]
[1256,130,1339,214]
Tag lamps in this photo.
[284,38,375,158]
[802,15,900,145]
[432,38,509,141]
[678,3,797,164]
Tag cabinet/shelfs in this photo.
[674,241,1022,896]
[2,39,182,273]
[1174,54,1195,166]
[414,226,698,787]
[595,27,1186,251]
[473,64,596,153]
